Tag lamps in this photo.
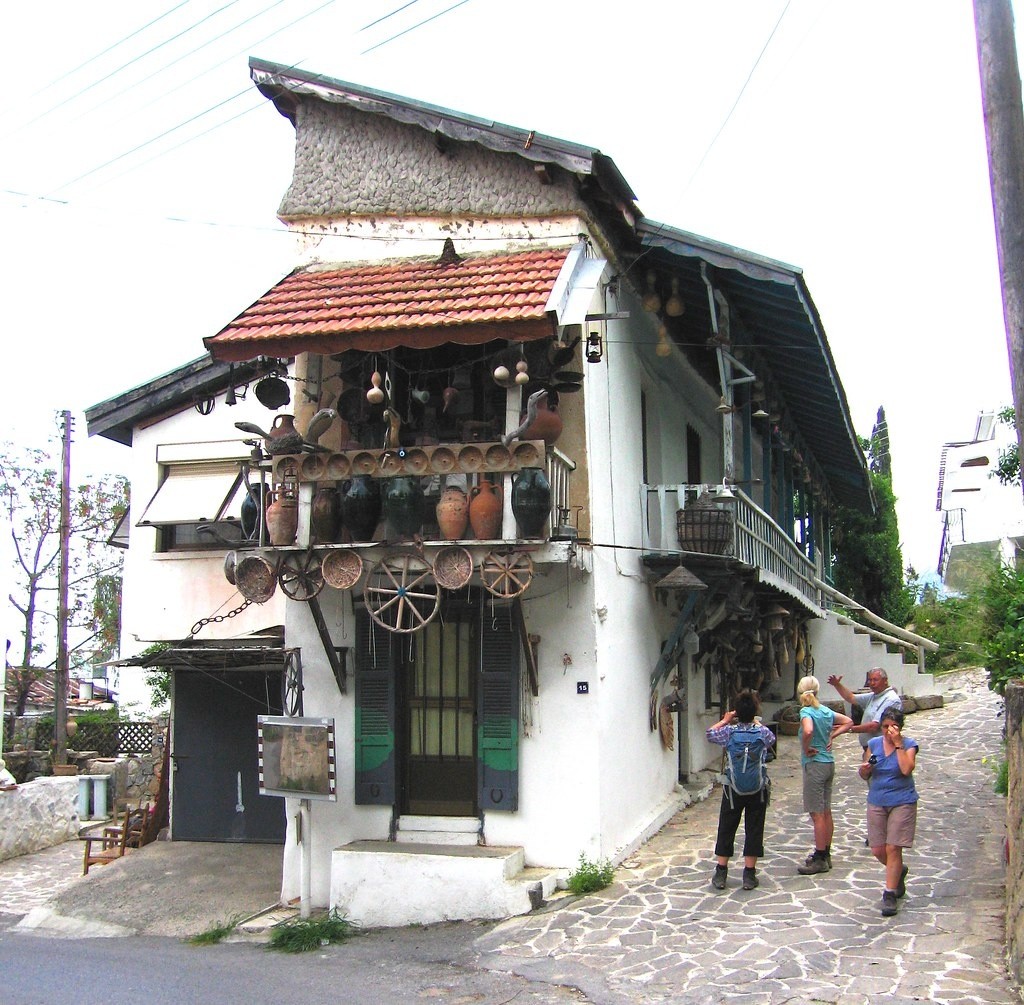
[731,399,769,418]
[586,319,602,362]
[712,478,739,503]
[715,396,731,413]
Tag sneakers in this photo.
[798,850,832,875]
[712,866,727,889]
[743,867,759,889]
[895,865,908,898]
[882,892,897,916]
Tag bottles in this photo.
[519,397,563,445]
[310,487,342,545]
[343,474,381,543]
[436,489,468,541]
[470,480,504,540]
[384,477,424,540]
[266,485,298,546]
[511,466,551,539]
[241,483,272,540]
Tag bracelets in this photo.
[895,745,904,749]
[721,721,727,726]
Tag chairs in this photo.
[78,802,149,876]
[102,799,141,850]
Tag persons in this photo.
[706,693,775,890]
[859,707,920,916]
[827,667,903,846]
[798,677,854,875]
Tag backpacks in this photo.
[720,724,767,809]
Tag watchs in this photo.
[848,728,853,733]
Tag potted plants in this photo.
[49,740,77,775]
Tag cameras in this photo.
[867,754,878,765]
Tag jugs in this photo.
[265,415,302,455]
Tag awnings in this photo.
[743,565,827,621]
